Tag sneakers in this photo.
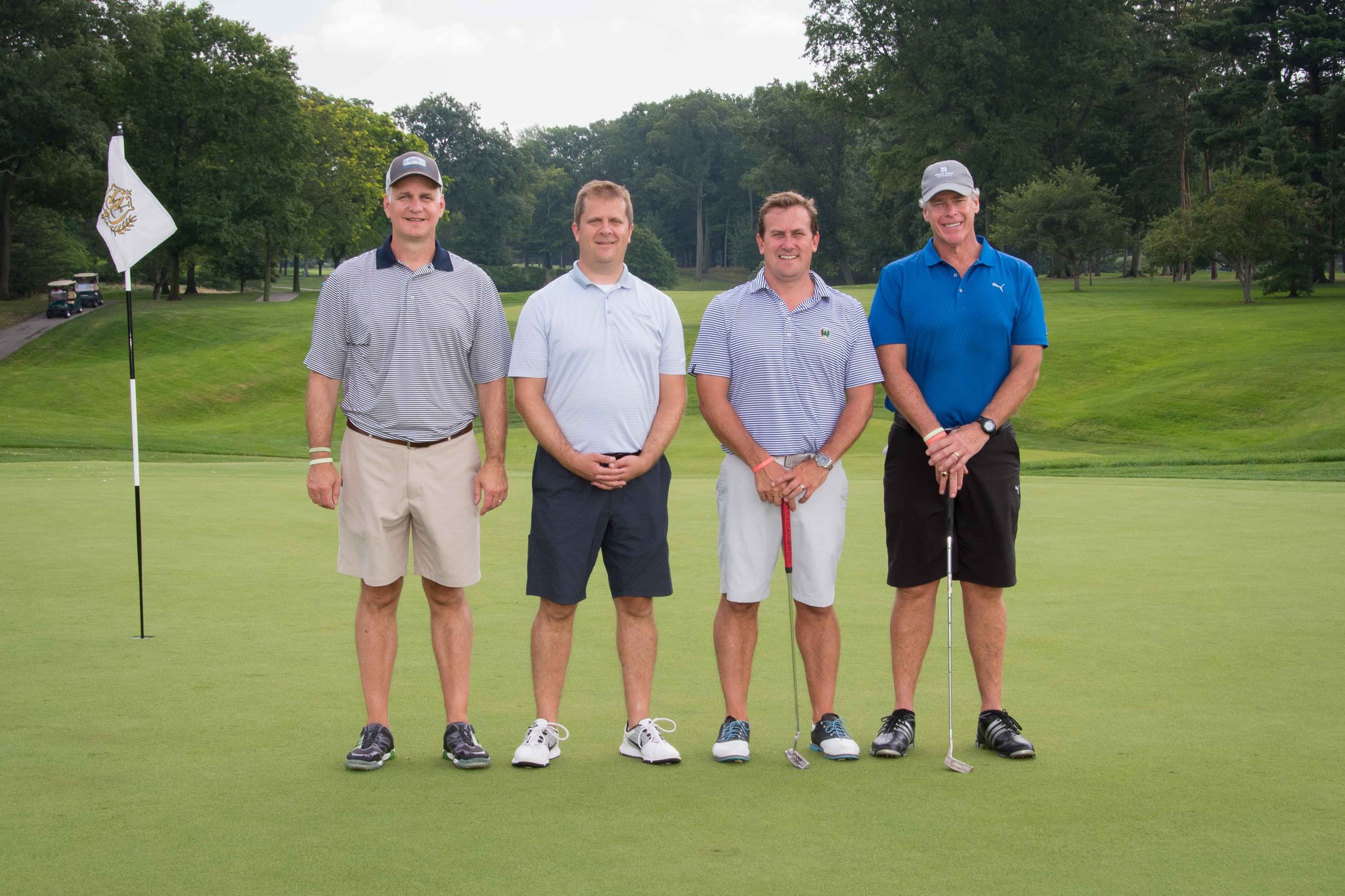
[511,718,570,768]
[870,709,916,757]
[809,713,860,760]
[712,715,751,762]
[442,723,491,769]
[346,723,395,770]
[619,718,682,764]
[975,709,1037,758]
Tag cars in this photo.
[1219,264,1233,272]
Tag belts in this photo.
[774,454,815,468]
[894,415,1011,437]
[346,417,473,449]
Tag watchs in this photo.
[814,453,833,471]
[976,416,997,438]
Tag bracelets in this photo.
[923,427,948,446]
[308,447,332,455]
[751,456,775,473]
[309,458,333,467]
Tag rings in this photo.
[799,484,805,491]
[498,499,503,504]
[953,451,961,459]
[940,471,948,477]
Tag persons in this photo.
[303,150,512,769]
[868,144,1049,758]
[508,179,685,769]
[687,189,885,763]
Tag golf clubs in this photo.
[779,500,811,771]
[943,486,974,774]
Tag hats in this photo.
[385,152,443,192]
[921,160,974,203]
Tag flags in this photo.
[96,134,178,274]
